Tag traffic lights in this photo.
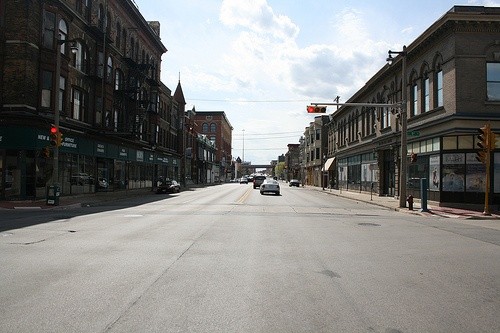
[307,105,327,114]
[41,146,50,159]
[475,150,487,162]
[477,126,488,146]
[49,127,58,149]
[410,153,418,163]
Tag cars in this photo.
[289,179,300,187]
[158,180,181,194]
[239,174,256,184]
[259,178,280,196]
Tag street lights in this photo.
[181,116,194,188]
[52,35,79,185]
[386,44,408,207]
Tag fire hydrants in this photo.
[406,194,415,210]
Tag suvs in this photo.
[253,176,266,189]
